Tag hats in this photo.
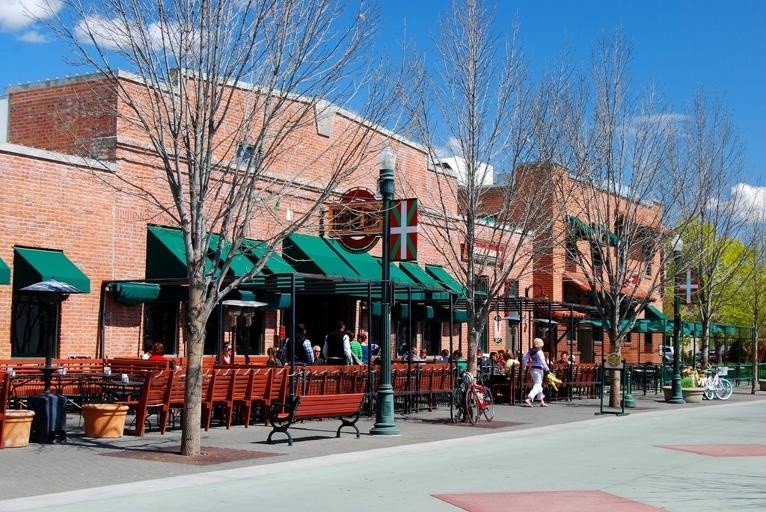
[534,337,544,348]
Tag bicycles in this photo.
[683,364,733,400]
[447,376,495,425]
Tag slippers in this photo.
[541,402,549,407]
[520,402,533,407]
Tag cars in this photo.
[662,346,675,366]
[695,350,731,363]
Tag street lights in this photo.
[368,144,403,434]
[667,234,687,404]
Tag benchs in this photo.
[0,357,111,405]
[267,389,367,445]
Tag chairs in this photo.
[118,367,289,434]
[600,356,750,397]
[492,364,595,404]
[289,364,455,395]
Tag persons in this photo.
[369,343,577,404]
[139,341,170,386]
[356,332,371,366]
[344,329,361,365]
[281,323,314,397]
[312,344,324,366]
[321,320,354,365]
[266,342,283,366]
[521,335,551,407]
[217,341,232,365]
